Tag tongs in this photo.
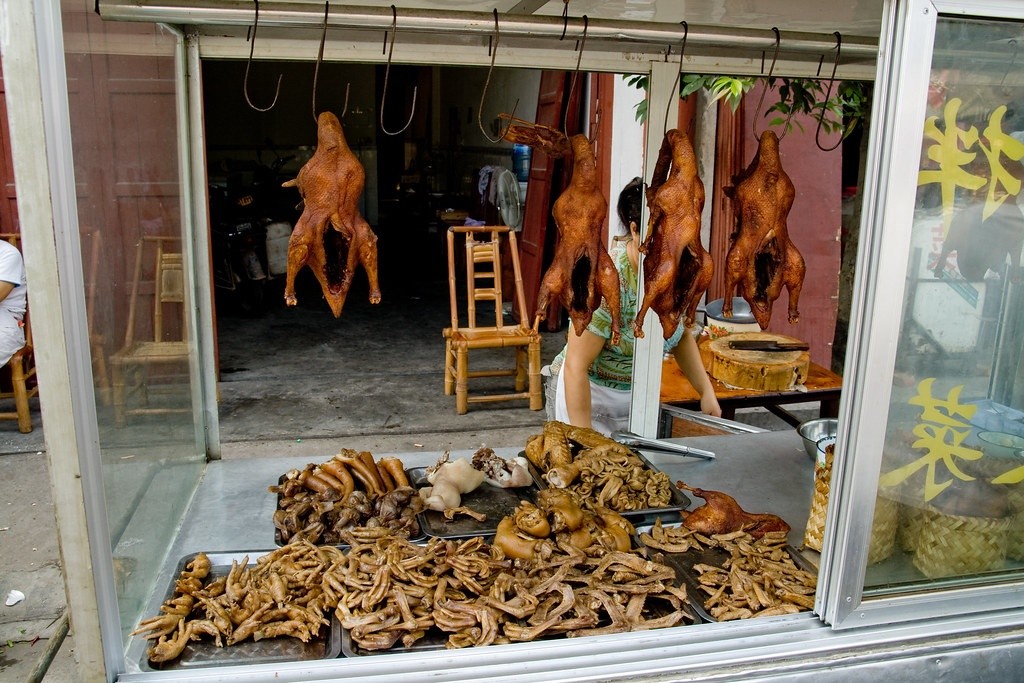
[611,430,715,461]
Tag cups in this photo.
[814,437,837,482]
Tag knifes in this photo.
[729,342,809,351]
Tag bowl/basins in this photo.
[977,431,1024,459]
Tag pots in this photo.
[696,297,761,339]
[762,400,838,462]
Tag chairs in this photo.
[443,226,542,415]
[0,231,220,433]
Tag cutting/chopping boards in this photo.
[699,332,809,390]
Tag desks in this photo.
[656,333,843,429]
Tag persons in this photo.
[0,239,26,369]
[540,175,722,445]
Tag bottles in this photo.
[514,144,530,182]
